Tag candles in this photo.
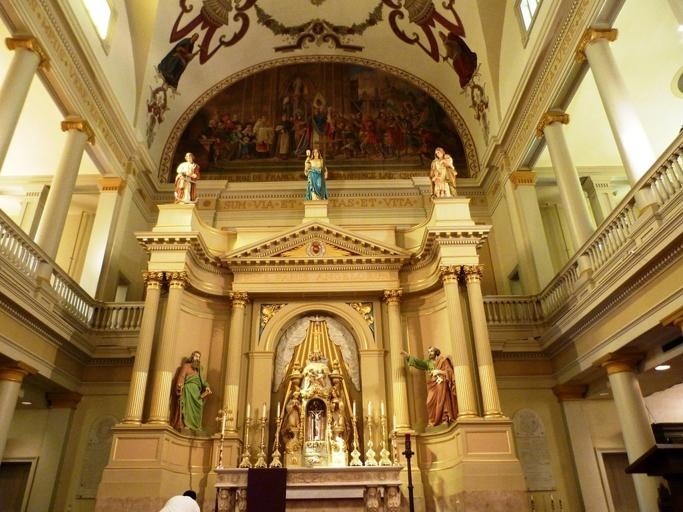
[263,402,267,417]
[277,402,281,418]
[367,401,372,415]
[380,402,385,415]
[221,415,226,434]
[353,400,356,418]
[246,402,251,418]
[392,416,397,429]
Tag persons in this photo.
[398,345,459,427]
[362,488,381,512]
[285,390,301,427]
[299,351,332,398]
[330,389,344,426]
[309,410,323,437]
[200,76,437,168]
[216,489,230,512]
[235,489,247,511]
[382,486,402,512]
[155,32,199,91]
[157,489,200,512]
[172,151,202,205]
[428,146,459,200]
[172,350,213,437]
[302,146,330,201]
[436,28,478,89]
